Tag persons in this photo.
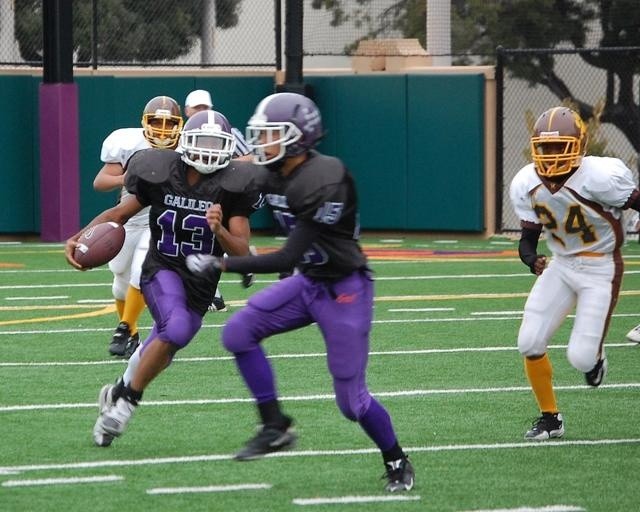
[62,107,267,447]
[508,104,639,443]
[187,91,417,494]
[183,87,257,315]
[91,94,188,362]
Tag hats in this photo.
[184,89,213,108]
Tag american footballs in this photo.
[75,221,125,269]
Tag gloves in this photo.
[186,253,217,276]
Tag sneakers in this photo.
[383,445,415,495]
[585,341,608,387]
[93,383,136,446]
[108,321,141,359]
[205,295,228,312]
[524,411,564,441]
[241,245,257,288]
[232,420,298,461]
[625,324,640,343]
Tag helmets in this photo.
[177,109,237,174]
[530,106,590,178]
[141,94,184,149]
[245,91,325,165]
